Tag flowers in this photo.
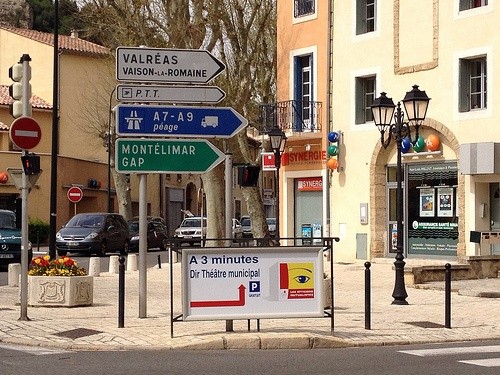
[27,255,89,277]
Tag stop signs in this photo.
[67,187,83,203]
[8,116,42,150]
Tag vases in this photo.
[17,274,94,308]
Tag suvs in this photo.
[0,209,33,269]
[173,216,207,247]
[56,211,131,257]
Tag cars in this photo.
[231,215,277,243]
[125,215,168,252]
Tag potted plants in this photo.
[323,272,332,310]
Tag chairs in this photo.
[197,221,205,227]
[246,220,250,225]
[95,216,103,226]
[186,221,194,227]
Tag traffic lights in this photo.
[21,153,40,176]
[238,164,260,187]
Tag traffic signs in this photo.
[115,46,227,85]
[114,137,226,174]
[116,102,249,139]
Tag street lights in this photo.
[370,84,433,304]
[268,125,288,246]
